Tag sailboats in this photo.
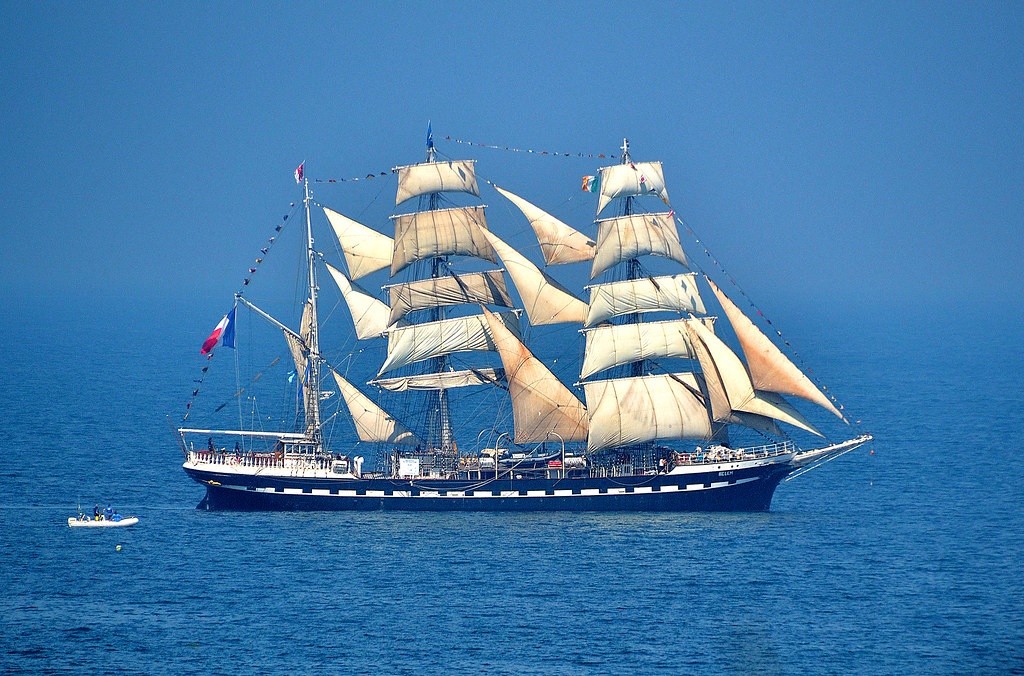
[175,116,874,515]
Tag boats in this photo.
[66,494,139,528]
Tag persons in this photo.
[208,436,240,454]
[414,443,423,452]
[94,504,125,520]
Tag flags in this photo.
[201,308,237,353]
[427,123,435,150]
[582,175,598,194]
[294,164,303,182]
